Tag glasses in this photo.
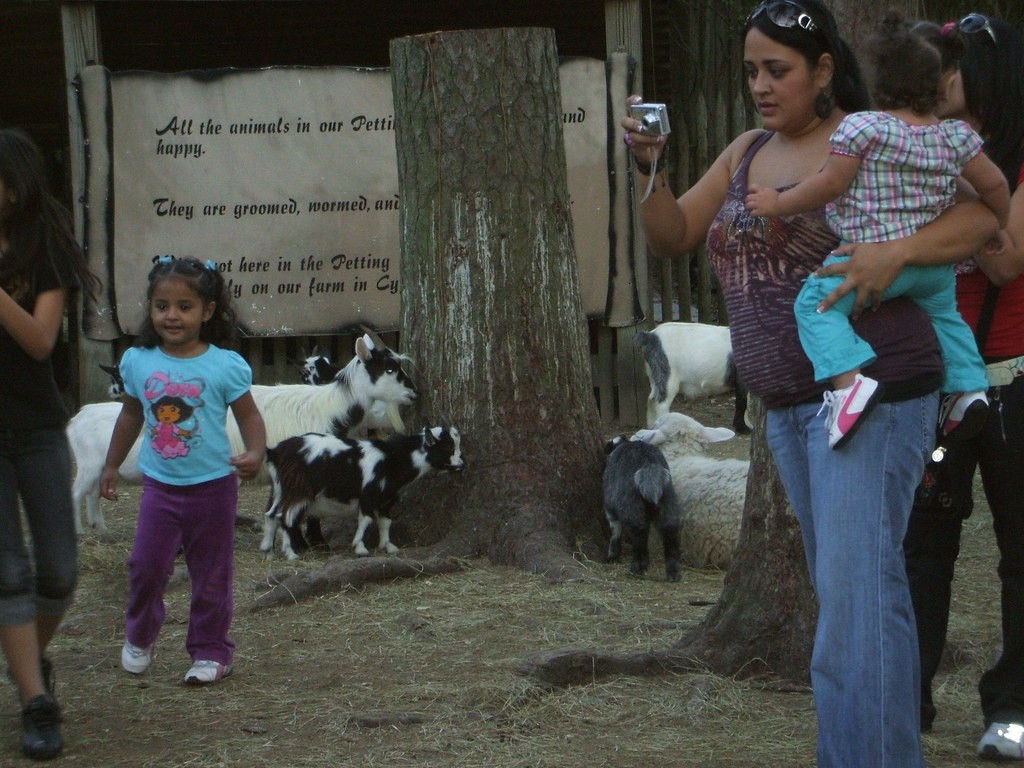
[960,13,996,45]
[738,1,819,38]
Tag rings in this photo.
[623,133,634,146]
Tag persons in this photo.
[621,0,1003,768]
[97,252,269,684]
[0,128,103,759]
[743,10,1012,450]
[904,13,1024,761]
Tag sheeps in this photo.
[601,435,683,583]
[65,401,148,535]
[276,345,339,385]
[633,320,755,436]
[99,364,126,399]
[225,324,420,486]
[259,416,464,562]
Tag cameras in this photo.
[631,103,671,138]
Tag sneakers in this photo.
[20,660,69,761]
[184,660,235,685]
[122,642,156,673]
[979,721,1024,760]
[940,391,991,441]
[822,372,883,451]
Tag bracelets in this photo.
[634,153,666,193]
